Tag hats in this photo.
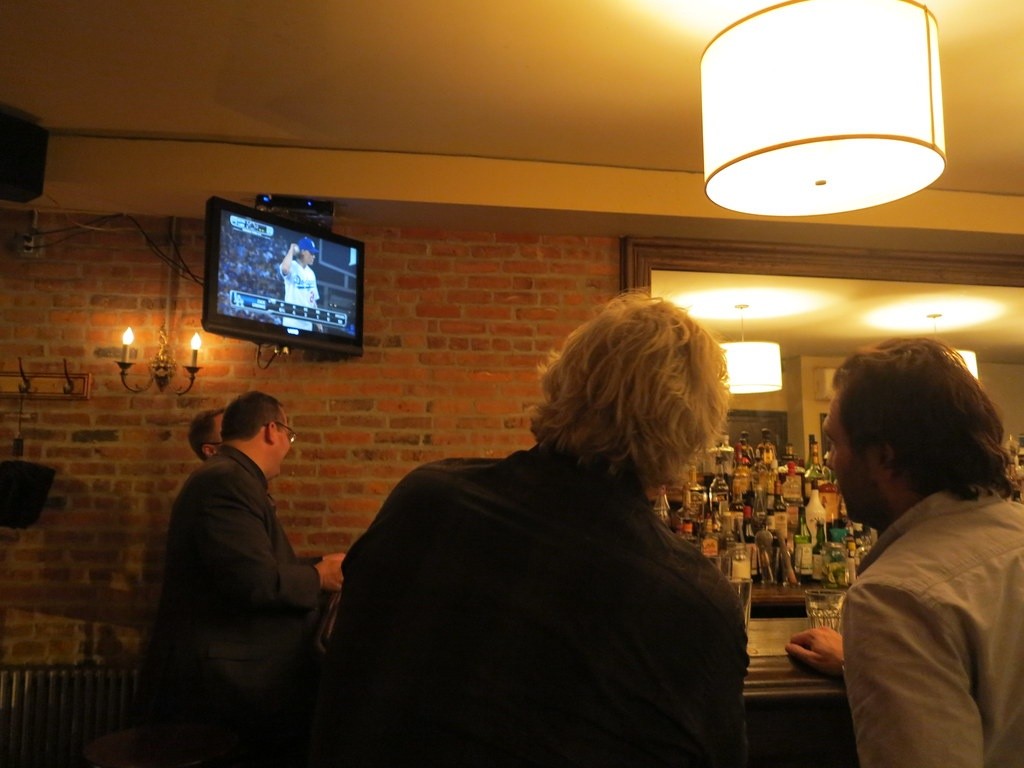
[298,237,319,257]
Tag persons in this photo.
[218,226,288,325]
[309,287,754,768]
[138,392,346,768]
[785,337,1024,768]
[278,235,323,309]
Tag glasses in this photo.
[265,421,298,447]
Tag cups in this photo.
[804,589,847,633]
[726,578,752,636]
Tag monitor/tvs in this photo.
[201,196,365,357]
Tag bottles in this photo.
[650,427,877,585]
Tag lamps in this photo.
[700,0,947,216]
[925,315,978,380]
[718,304,782,393]
[114,326,204,395]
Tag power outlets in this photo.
[14,231,50,259]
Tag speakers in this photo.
[0,113,49,203]
[0,460,56,530]
